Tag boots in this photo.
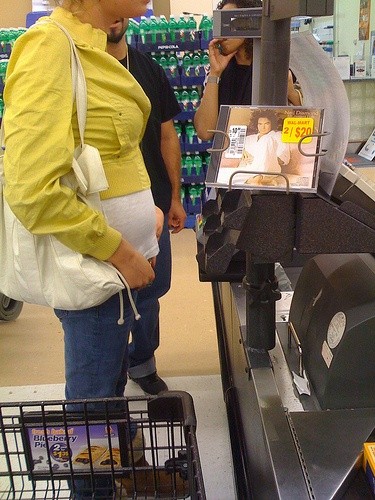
[112,433,197,498]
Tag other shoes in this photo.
[128,363,168,396]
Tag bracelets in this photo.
[206,76,220,84]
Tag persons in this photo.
[104,16,187,396]
[0,0,165,499]
[234,109,291,186]
[194,0,306,142]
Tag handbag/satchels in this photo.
[0,21,141,325]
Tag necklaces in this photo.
[126,44,130,71]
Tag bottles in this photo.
[0,27,30,117]
[125,14,214,206]
